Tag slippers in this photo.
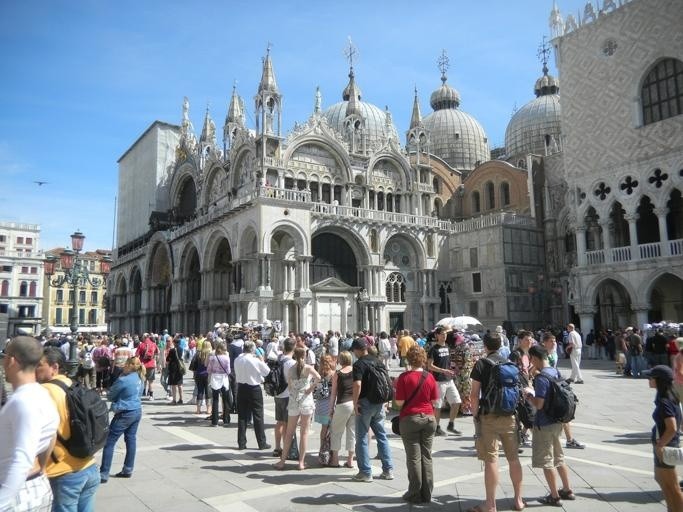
[219,414,223,419]
[205,415,212,420]
[343,462,354,468]
[298,463,309,469]
[324,463,338,467]
[464,504,483,511]
[272,463,282,469]
[515,499,526,510]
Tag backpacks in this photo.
[359,358,394,405]
[263,354,290,396]
[45,379,110,458]
[82,346,96,370]
[479,358,520,415]
[535,368,579,424]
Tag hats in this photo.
[121,338,128,345]
[675,337,683,351]
[347,337,367,353]
[642,364,673,381]
[141,332,148,338]
[495,325,502,332]
[625,326,633,332]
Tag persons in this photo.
[362,329,374,346]
[641,364,683,512]
[426,327,463,437]
[180,328,205,361]
[128,334,143,355]
[339,330,354,352]
[214,322,226,342]
[399,329,414,372]
[272,346,322,471]
[162,328,170,345]
[111,338,132,378]
[79,339,97,389]
[166,336,184,405]
[233,340,272,451]
[266,337,279,361]
[207,341,231,427]
[672,336,683,408]
[526,345,576,507]
[325,330,339,356]
[272,337,297,457]
[205,337,224,421]
[160,336,174,400]
[623,327,634,376]
[624,327,643,377]
[495,325,510,347]
[395,345,440,503]
[92,340,111,387]
[378,331,391,371]
[293,334,316,368]
[36,347,102,512]
[469,332,525,512]
[100,356,146,484]
[508,331,533,453]
[347,338,394,483]
[587,329,597,360]
[58,335,73,361]
[135,333,160,401]
[225,333,243,414]
[191,340,212,414]
[0,335,61,512]
[606,328,615,361]
[614,331,627,362]
[565,323,585,384]
[652,328,670,365]
[543,334,559,368]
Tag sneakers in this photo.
[519,430,528,444]
[434,426,447,436]
[167,400,175,405]
[176,399,182,404]
[446,424,463,435]
[166,392,169,399]
[352,471,372,481]
[168,396,172,400]
[565,439,585,449]
[378,468,392,478]
[186,399,196,404]
[273,448,282,456]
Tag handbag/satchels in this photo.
[96,346,111,369]
[390,369,427,436]
[654,423,683,467]
[318,416,333,465]
[287,431,299,459]
[191,353,197,370]
[628,336,639,356]
[267,341,278,361]
[215,353,234,386]
[175,348,186,374]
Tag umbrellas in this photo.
[435,314,483,327]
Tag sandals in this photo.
[558,488,575,499]
[115,471,131,477]
[536,494,563,507]
[100,478,106,483]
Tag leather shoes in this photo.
[259,443,271,450]
[239,445,246,450]
[575,380,583,383]
[566,378,573,382]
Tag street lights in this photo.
[42,228,113,378]
[527,270,563,328]
